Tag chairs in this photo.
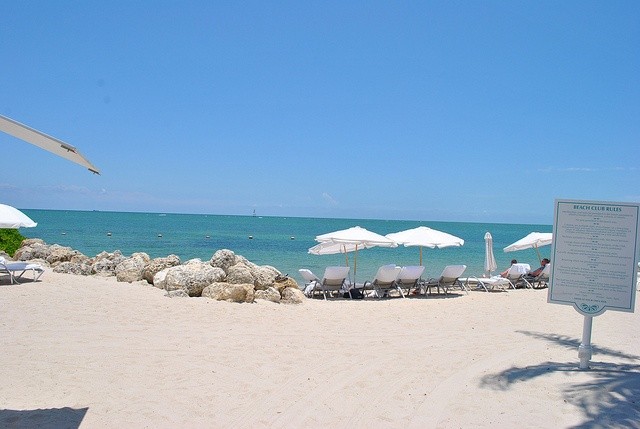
[458,278,512,292]
[298,269,320,291]
[0,262,45,285]
[417,265,469,298]
[304,266,353,300]
[350,264,405,300]
[395,266,428,298]
[505,264,536,292]
[523,263,550,289]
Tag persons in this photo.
[482,259,517,279]
[524,258,550,277]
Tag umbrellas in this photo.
[483,232,498,279]
[308,242,376,286]
[0,204,38,229]
[385,225,465,281]
[503,231,553,266]
[316,226,399,288]
